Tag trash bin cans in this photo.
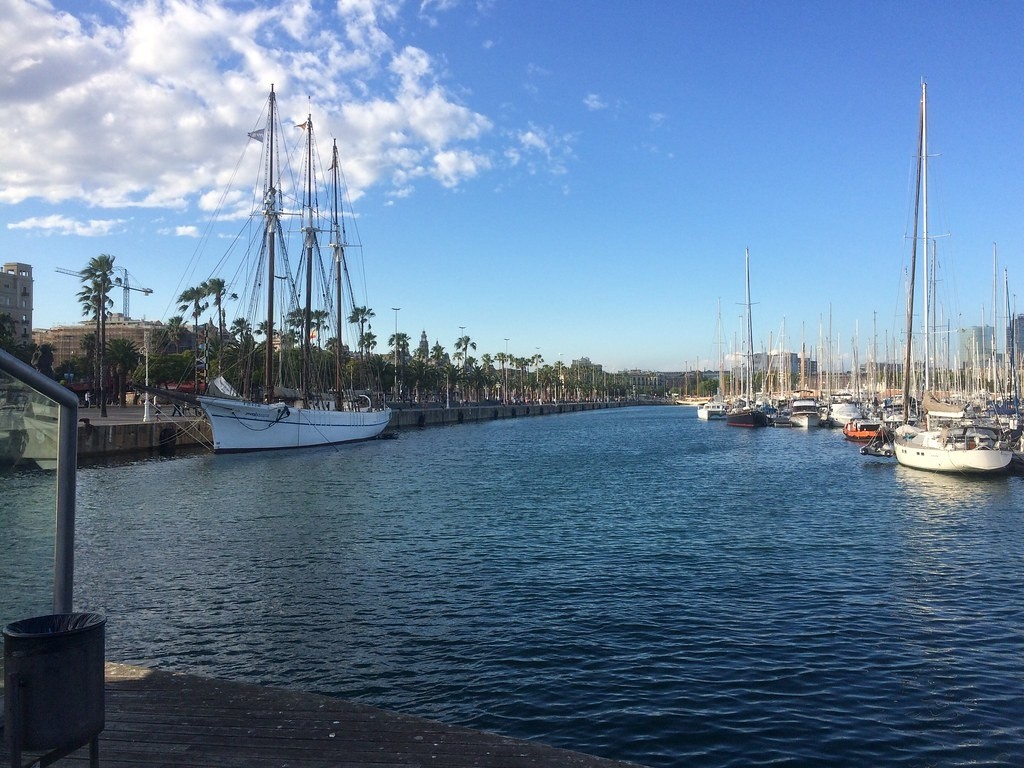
[1,610,114,750]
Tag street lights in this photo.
[536,347,541,402]
[459,326,466,361]
[391,308,401,401]
[559,353,565,399]
[504,338,510,404]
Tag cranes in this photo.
[55,267,154,320]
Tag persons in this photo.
[184,401,191,417]
[501,392,629,407]
[154,402,162,422]
[83,390,95,408]
[171,403,183,417]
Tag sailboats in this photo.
[130,85,395,455]
[676,78,1023,476]
